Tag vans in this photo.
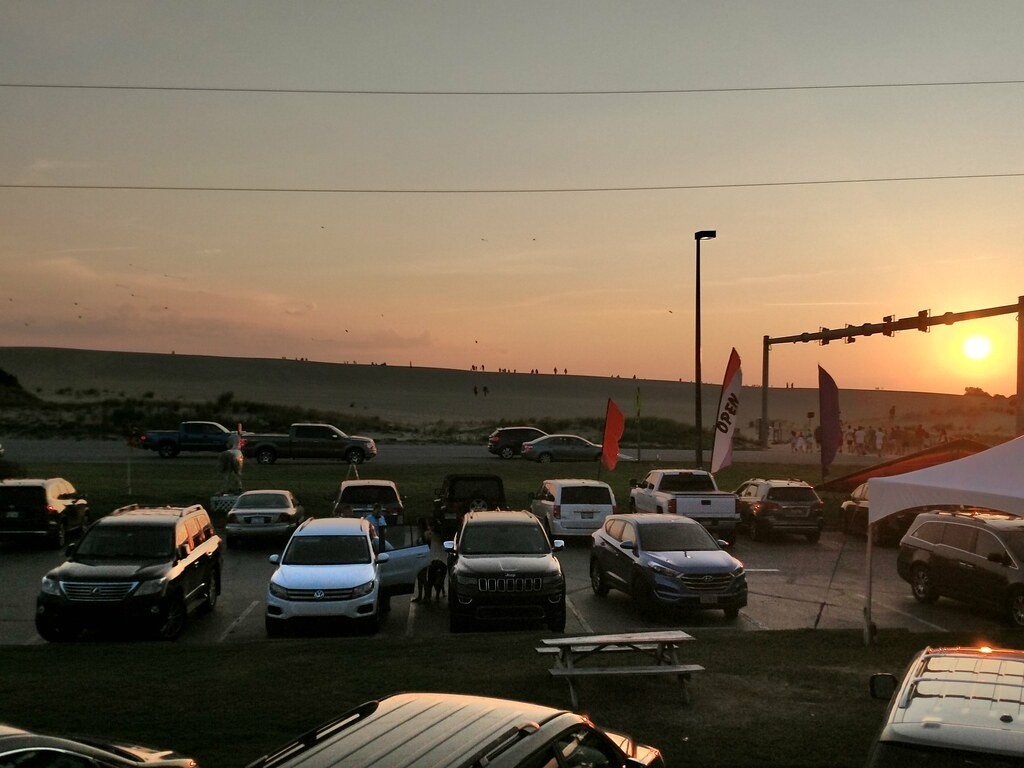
[528,480,618,544]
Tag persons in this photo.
[365,503,388,536]
[789,424,949,457]
[412,518,448,601]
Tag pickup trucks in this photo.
[240,423,377,466]
[127,420,234,460]
[627,469,743,548]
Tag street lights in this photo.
[693,228,717,470]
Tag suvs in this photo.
[33,504,225,645]
[897,510,1024,631]
[245,690,667,768]
[265,515,433,636]
[866,646,1024,768]
[332,481,408,547]
[443,507,567,629]
[436,472,506,540]
[589,514,749,620]
[732,477,826,543]
[488,427,549,460]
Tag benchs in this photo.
[535,644,707,707]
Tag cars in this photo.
[225,489,305,548]
[838,481,927,546]
[0,720,200,768]
[0,479,94,551]
[519,434,604,463]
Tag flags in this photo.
[820,368,842,465]
[710,347,742,473]
[601,400,625,472]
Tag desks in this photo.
[539,630,696,711]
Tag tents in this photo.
[814,438,993,490]
[865,433,1024,649]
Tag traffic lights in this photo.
[882,315,895,337]
[844,323,856,344]
